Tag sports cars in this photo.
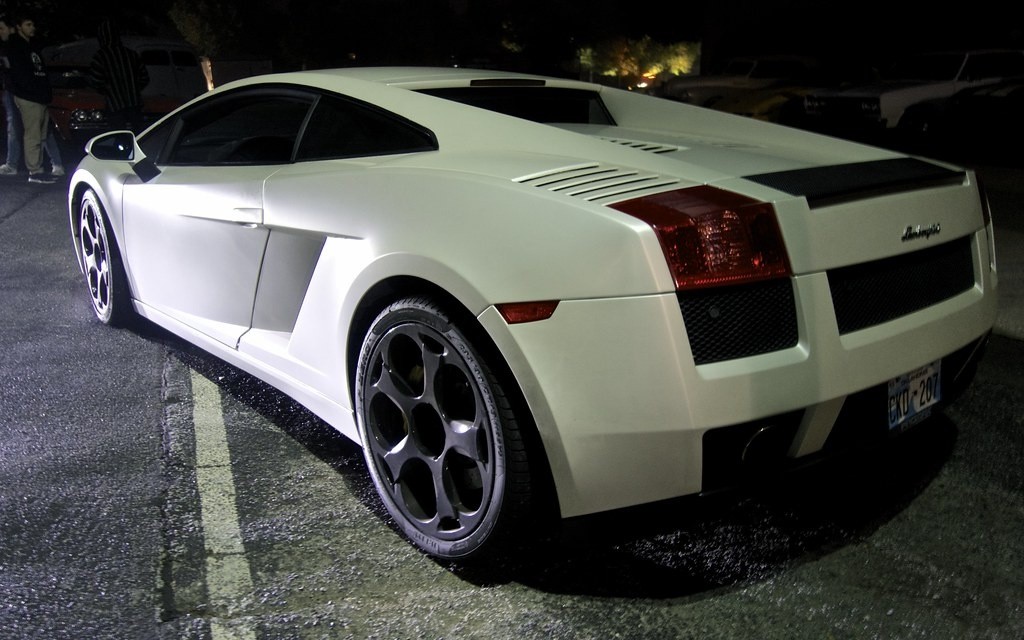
[66,66,998,563]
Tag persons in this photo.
[88,20,150,135]
[0,13,65,183]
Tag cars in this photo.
[0,39,197,142]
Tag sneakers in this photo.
[52,165,66,177]
[28,173,55,183]
[0,164,17,175]
